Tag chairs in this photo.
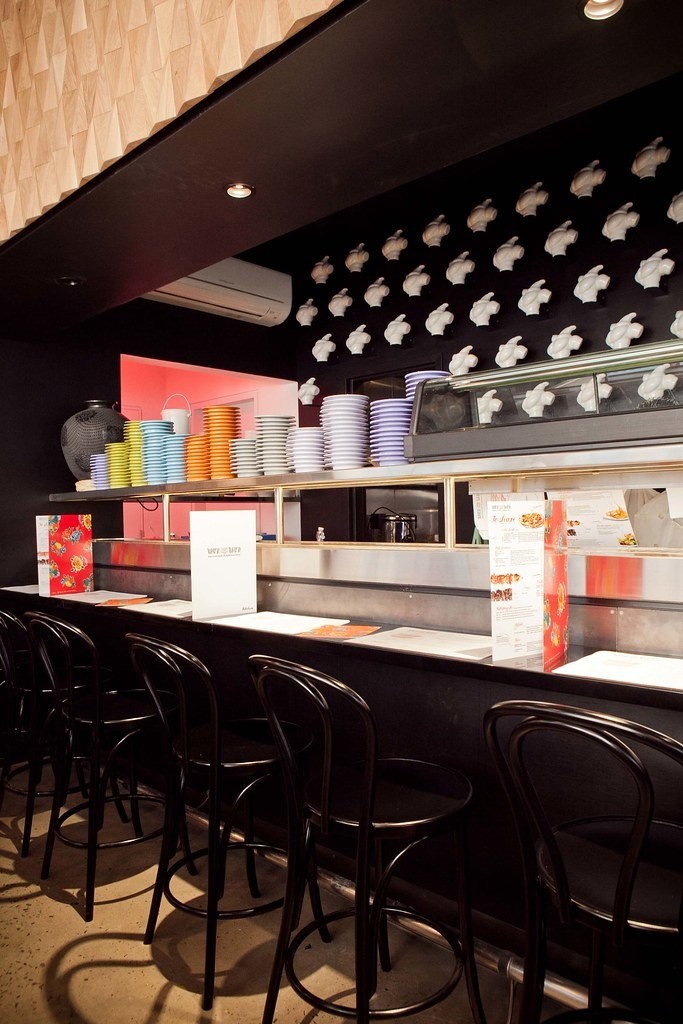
[0,610,130,858]
[122,632,333,1011]
[248,653,488,1024]
[22,609,198,920]
[482,700,683,1024]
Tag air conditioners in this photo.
[139,256,292,327]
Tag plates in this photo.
[619,541,638,547]
[90,370,368,492]
[603,512,628,520]
[370,371,451,466]
[519,512,545,528]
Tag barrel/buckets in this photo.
[370,512,417,542]
[161,394,191,434]
